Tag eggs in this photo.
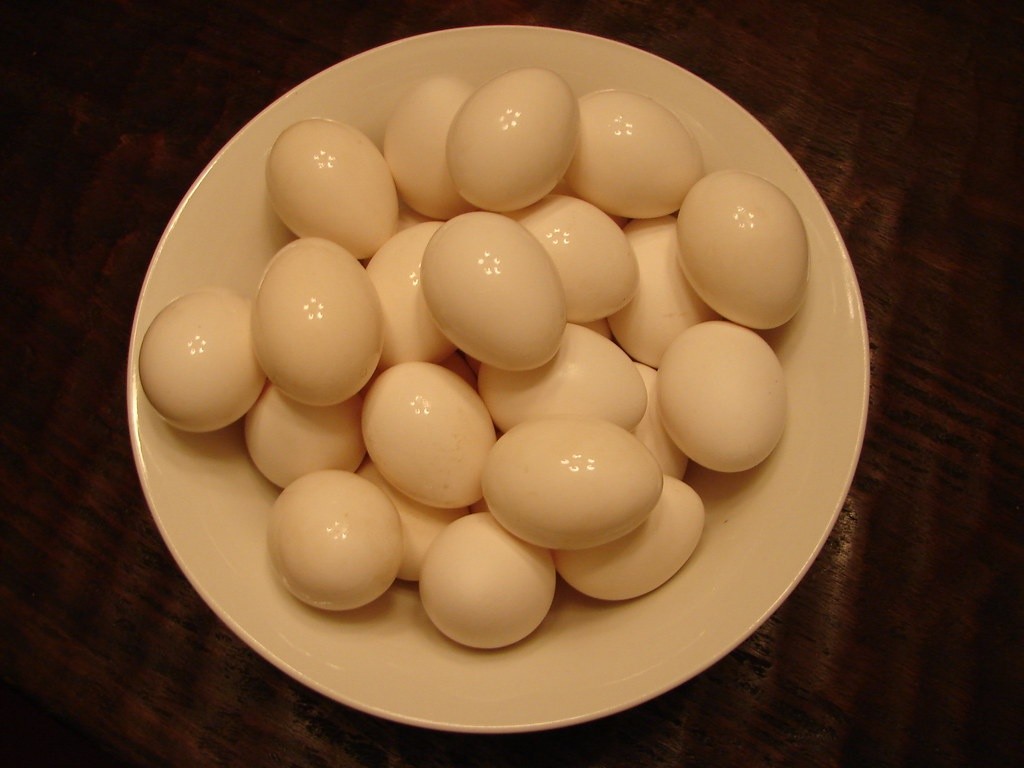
[140,66,811,651]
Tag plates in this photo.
[128,26,871,734]
